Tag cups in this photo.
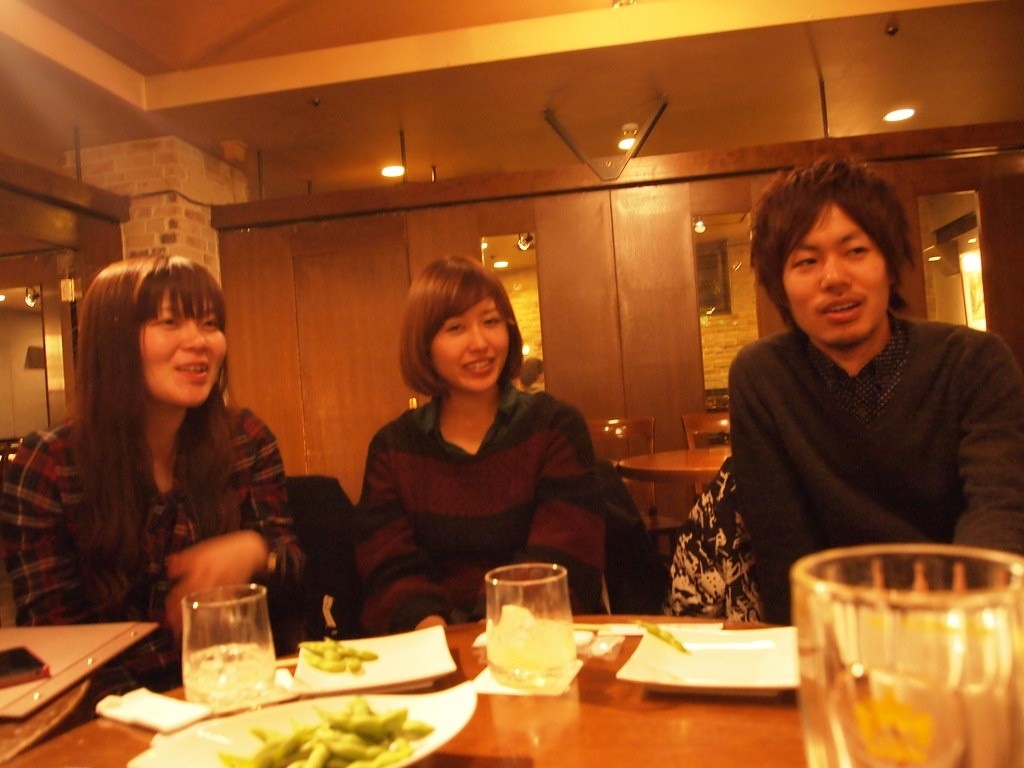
[486,561,577,687]
[180,584,277,709]
[788,542,1024,768]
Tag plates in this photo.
[290,624,459,698]
[615,625,800,698]
[125,681,476,768]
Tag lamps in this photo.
[518,232,533,250]
[25,283,40,307]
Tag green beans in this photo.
[220,691,436,768]
[636,621,690,653]
[299,637,378,672]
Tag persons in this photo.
[729,156,1024,625]
[0,258,305,704]
[352,257,604,636]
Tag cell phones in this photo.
[0,646,50,689]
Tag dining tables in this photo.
[4,614,804,768]
[614,443,730,503]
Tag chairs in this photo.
[267,478,365,652]
[682,410,730,498]
[587,418,688,559]
